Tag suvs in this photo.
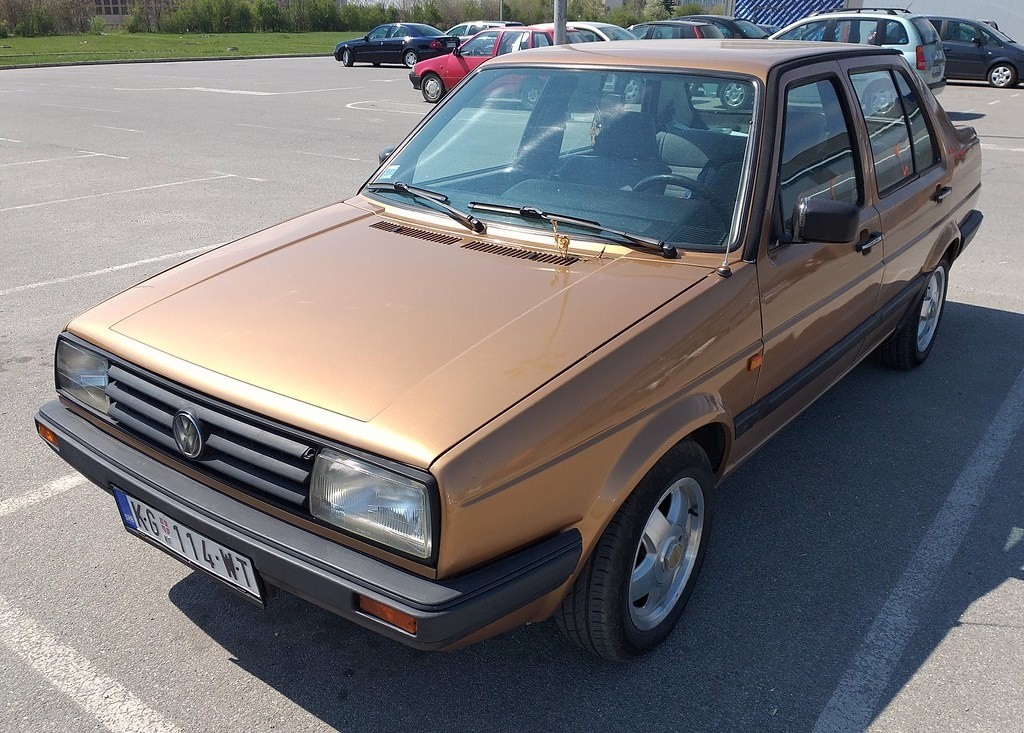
[718,7,947,119]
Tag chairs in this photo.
[553,111,672,194]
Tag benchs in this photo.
[657,129,749,198]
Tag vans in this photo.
[444,20,525,51]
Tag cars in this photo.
[511,21,646,105]
[867,15,1024,89]
[625,21,725,96]
[34,37,984,670]
[757,24,783,36]
[671,14,769,40]
[409,27,607,112]
[333,22,461,69]
